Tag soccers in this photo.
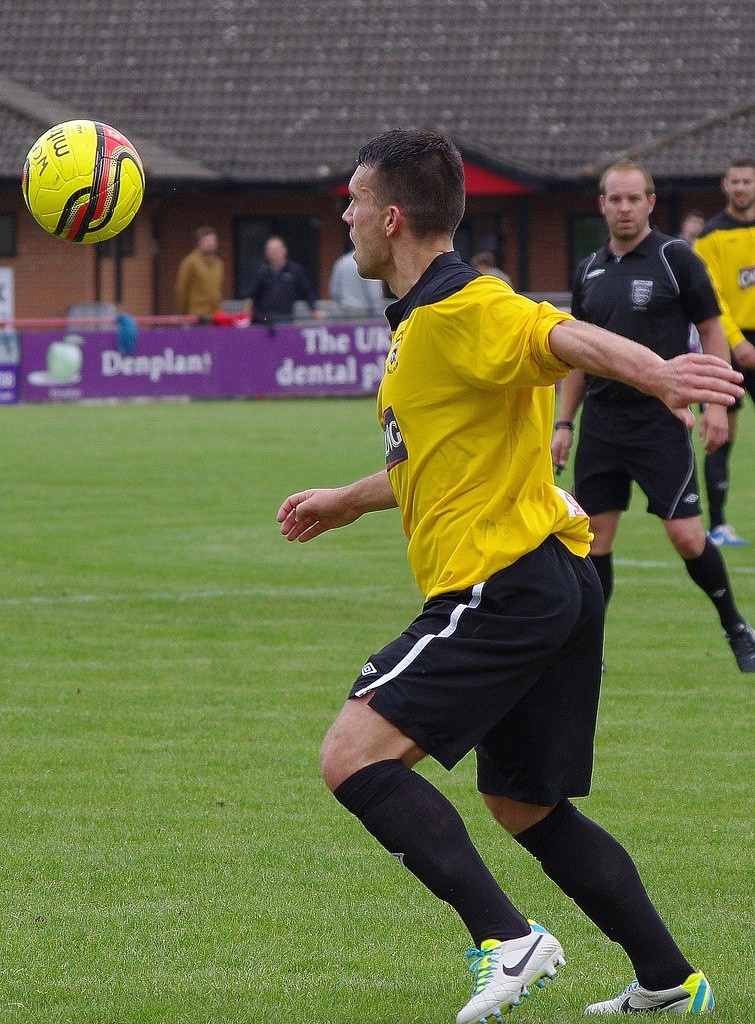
[17,117,149,247]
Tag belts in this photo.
[589,391,655,403]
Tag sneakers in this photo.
[725,621,755,672]
[457,918,565,1024]
[585,970,715,1016]
[706,524,749,546]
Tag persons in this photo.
[171,224,227,326]
[675,210,709,413]
[242,236,325,327]
[276,129,746,1024]
[550,157,755,674]
[328,245,384,318]
[689,157,755,548]
[470,250,513,288]
